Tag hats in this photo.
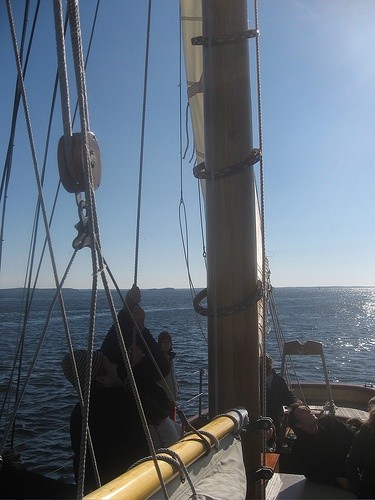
[61,350,103,393]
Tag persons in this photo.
[347,397,375,495]
[61,284,177,487]
[286,404,357,488]
[266,356,303,436]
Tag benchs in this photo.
[284,403,369,423]
